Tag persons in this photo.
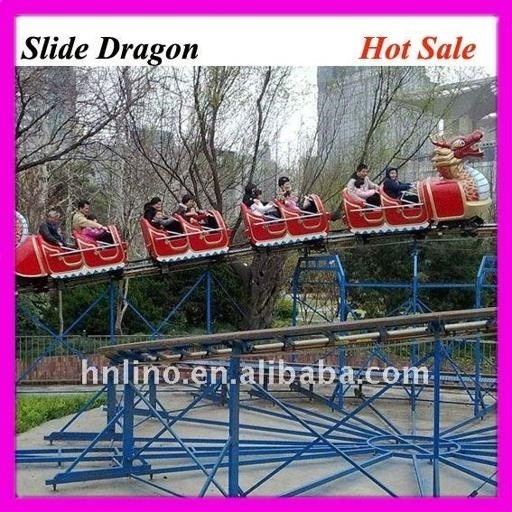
[37,200,112,250]
[144,195,219,233]
[243,176,316,221]
[345,164,419,209]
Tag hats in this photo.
[278,176,290,186]
